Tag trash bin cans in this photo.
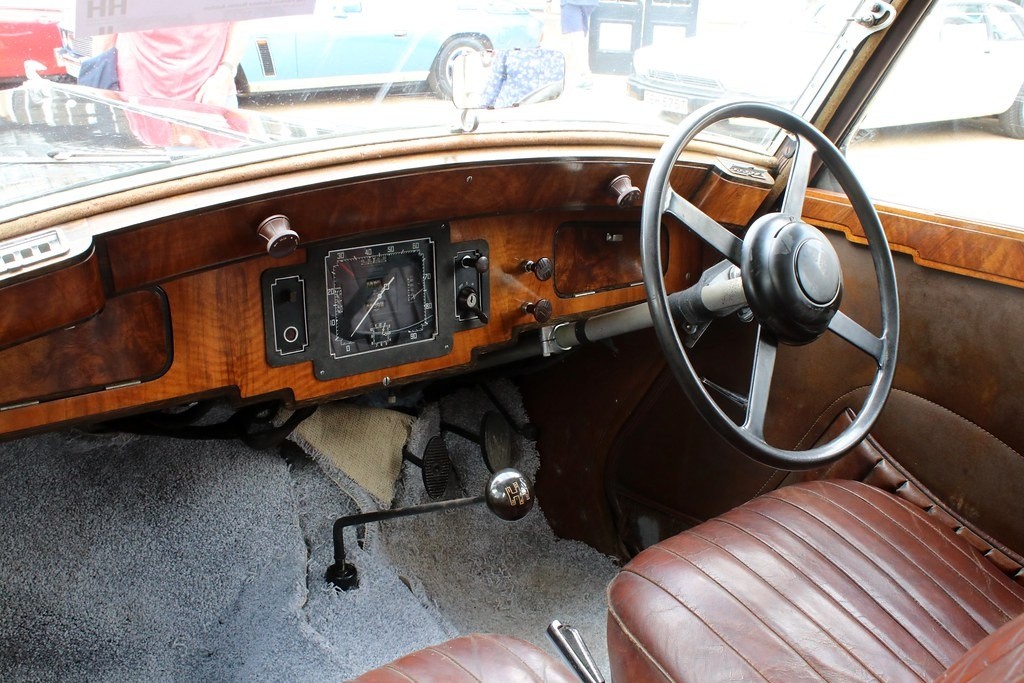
[588,0,644,74]
[560,0,600,34]
[643,0,699,47]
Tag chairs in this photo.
[606,481,1024,683]
[342,635,581,683]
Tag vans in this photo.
[0,21,66,82]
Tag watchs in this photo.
[219,59,239,76]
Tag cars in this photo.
[79,48,119,90]
[229,0,538,103]
[627,0,1024,141]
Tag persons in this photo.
[122,93,249,150]
[559,0,598,91]
[90,22,249,107]
